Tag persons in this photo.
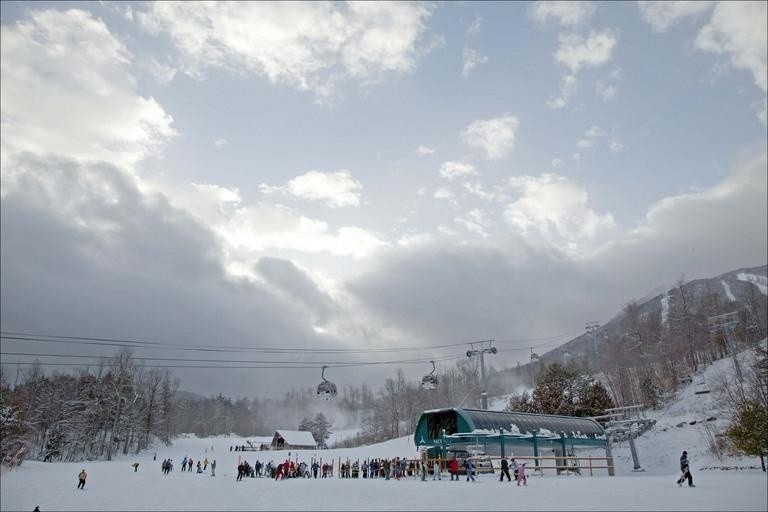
[496,457,511,482]
[160,456,216,477]
[76,469,87,490]
[515,464,527,487]
[508,458,525,480]
[235,456,477,484]
[131,463,139,473]
[675,450,696,489]
